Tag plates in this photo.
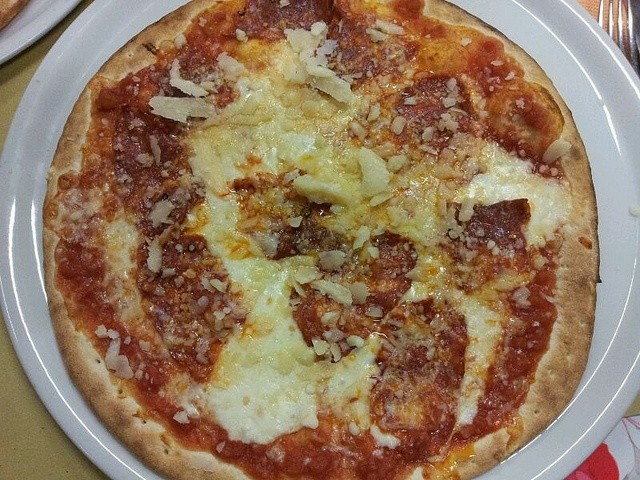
[0,1,83,67]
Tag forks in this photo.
[597,1,640,78]
